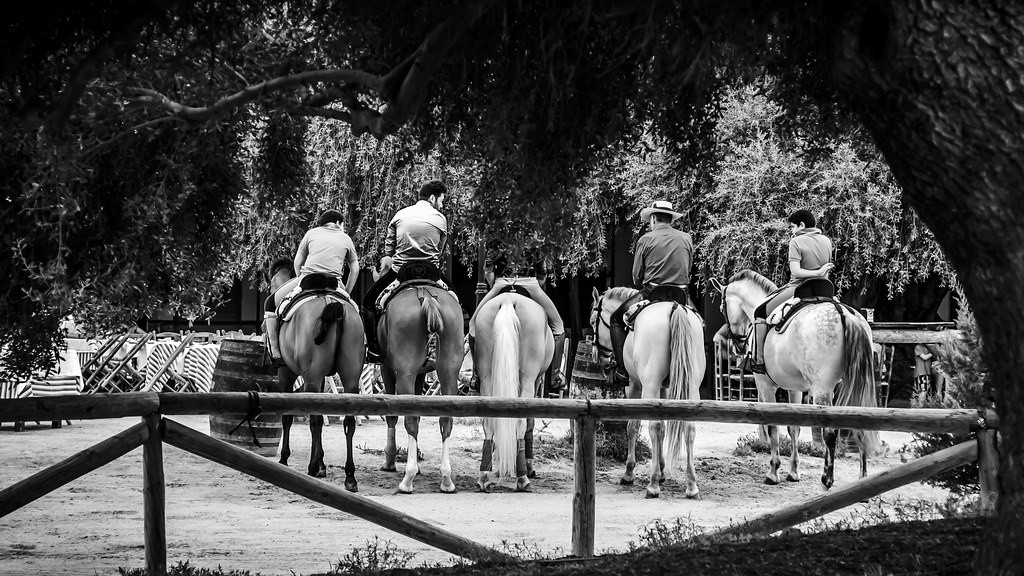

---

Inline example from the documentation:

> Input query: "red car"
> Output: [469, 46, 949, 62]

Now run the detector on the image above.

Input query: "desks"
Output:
[1, 337, 221, 394]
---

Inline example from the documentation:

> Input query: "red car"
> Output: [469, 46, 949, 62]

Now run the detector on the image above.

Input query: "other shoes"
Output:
[366, 350, 381, 363]
[270, 358, 287, 367]
[730, 361, 765, 374]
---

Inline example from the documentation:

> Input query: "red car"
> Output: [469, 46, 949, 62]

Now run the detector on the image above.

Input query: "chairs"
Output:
[76, 326, 256, 393]
[715, 340, 758, 402]
[293, 333, 475, 426]
[873, 344, 895, 408]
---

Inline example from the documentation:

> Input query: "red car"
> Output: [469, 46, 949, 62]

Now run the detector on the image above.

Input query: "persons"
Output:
[744, 209, 836, 373]
[911, 327, 933, 407]
[605, 201, 694, 386]
[263, 210, 360, 366]
[362, 181, 452, 363]
[872, 343, 888, 380]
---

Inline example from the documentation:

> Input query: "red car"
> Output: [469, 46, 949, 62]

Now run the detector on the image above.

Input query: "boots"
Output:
[605, 324, 629, 386]
[468, 334, 480, 390]
[551, 332, 567, 395]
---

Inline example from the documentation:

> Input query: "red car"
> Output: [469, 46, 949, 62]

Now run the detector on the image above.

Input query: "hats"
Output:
[640, 201, 683, 221]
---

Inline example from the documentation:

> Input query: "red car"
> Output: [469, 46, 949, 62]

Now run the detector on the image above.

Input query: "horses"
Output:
[709, 269, 875, 503]
[473, 292, 554, 492]
[264, 259, 366, 492]
[590, 287, 706, 500]
[376, 281, 464, 493]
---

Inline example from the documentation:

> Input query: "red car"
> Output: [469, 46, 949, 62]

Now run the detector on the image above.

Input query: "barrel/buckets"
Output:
[569, 340, 628, 440]
[208, 339, 291, 458]
